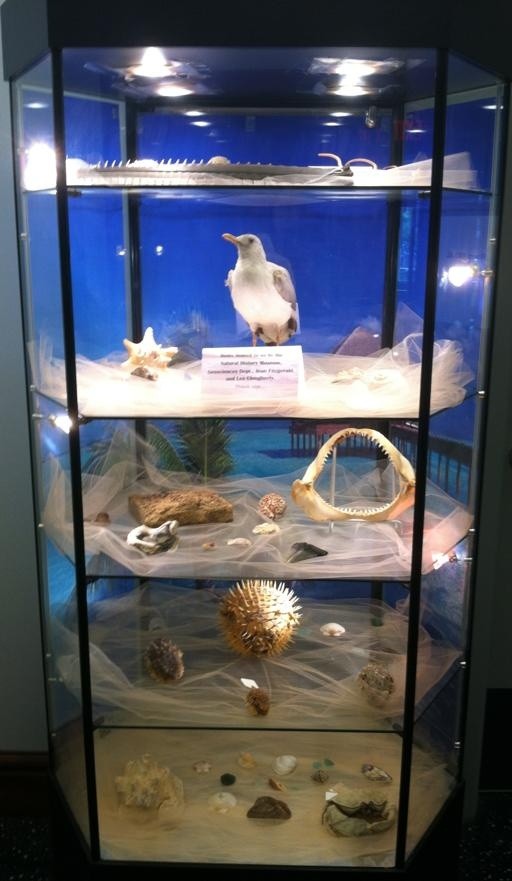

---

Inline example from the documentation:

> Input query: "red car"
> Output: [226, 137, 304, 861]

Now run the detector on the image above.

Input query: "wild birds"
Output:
[222, 231, 301, 346]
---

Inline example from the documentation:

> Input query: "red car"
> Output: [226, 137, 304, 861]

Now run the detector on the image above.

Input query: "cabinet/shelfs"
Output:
[0, 0, 512, 881]
